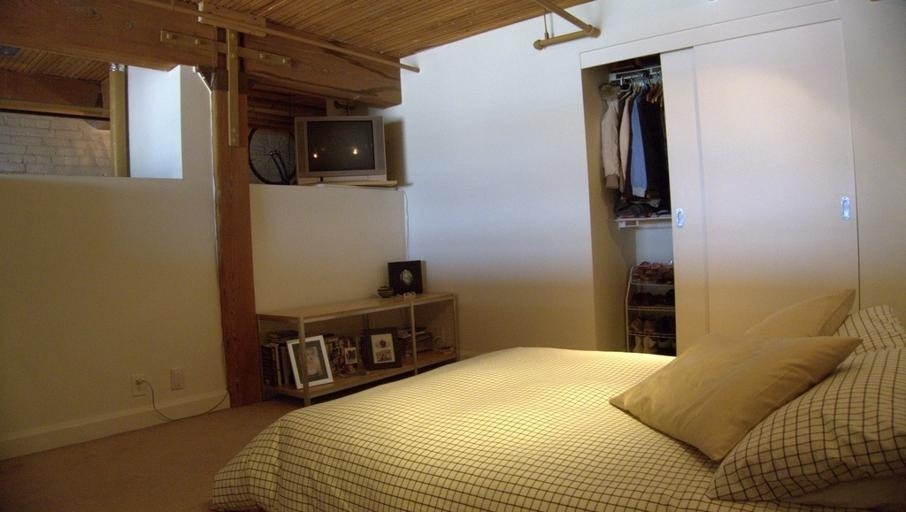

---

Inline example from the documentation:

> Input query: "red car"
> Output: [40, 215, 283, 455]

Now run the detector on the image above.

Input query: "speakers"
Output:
[387, 260, 423, 295]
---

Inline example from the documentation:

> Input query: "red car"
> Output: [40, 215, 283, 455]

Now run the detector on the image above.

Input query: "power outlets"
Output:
[132, 373, 147, 396]
[171, 368, 185, 390]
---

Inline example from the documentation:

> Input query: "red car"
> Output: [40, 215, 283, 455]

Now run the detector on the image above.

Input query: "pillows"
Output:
[784, 476, 906, 508]
[745, 289, 856, 336]
[706, 349, 906, 501]
[610, 330, 863, 462]
[831, 305, 905, 358]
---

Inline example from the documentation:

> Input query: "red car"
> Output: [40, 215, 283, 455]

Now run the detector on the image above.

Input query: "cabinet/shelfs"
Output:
[625, 266, 676, 351]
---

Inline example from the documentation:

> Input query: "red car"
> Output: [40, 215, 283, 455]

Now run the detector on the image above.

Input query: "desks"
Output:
[257, 292, 460, 407]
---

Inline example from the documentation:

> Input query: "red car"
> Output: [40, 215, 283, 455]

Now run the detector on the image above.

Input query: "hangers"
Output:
[611, 71, 663, 107]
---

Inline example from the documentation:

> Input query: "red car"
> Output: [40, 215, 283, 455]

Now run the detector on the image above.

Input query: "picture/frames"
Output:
[287, 336, 333, 389]
[365, 327, 401, 371]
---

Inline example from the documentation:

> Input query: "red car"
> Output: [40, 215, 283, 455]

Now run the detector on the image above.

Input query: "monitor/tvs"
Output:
[294, 116, 388, 183]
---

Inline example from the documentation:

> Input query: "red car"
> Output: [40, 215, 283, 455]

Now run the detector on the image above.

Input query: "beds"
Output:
[213, 347, 906, 512]
